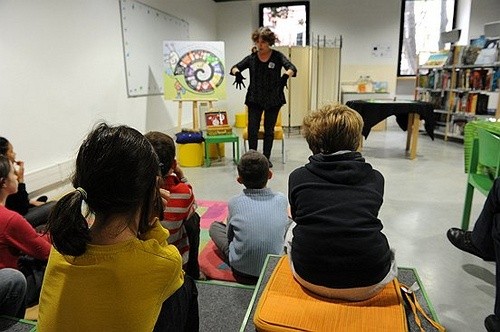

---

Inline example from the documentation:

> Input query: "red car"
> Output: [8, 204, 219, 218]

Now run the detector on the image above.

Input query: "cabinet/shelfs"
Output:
[414, 46, 500, 141]
[342, 84, 391, 131]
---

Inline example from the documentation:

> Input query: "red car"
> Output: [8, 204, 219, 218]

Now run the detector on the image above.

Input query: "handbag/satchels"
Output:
[175, 130, 205, 144]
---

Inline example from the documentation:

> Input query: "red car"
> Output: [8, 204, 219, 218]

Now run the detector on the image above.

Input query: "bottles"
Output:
[365, 75, 373, 92]
[358, 76, 364, 92]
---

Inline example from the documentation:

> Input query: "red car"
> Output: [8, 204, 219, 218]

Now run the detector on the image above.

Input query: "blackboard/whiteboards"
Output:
[119, 0, 190, 98]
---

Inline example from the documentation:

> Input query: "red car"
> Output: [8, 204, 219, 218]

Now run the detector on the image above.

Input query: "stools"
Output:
[235, 113, 246, 128]
[177, 141, 206, 167]
[203, 131, 240, 167]
[205, 142, 225, 158]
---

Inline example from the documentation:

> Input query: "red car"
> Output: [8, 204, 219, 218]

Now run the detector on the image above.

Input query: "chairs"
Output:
[461, 128, 500, 231]
[242, 104, 286, 164]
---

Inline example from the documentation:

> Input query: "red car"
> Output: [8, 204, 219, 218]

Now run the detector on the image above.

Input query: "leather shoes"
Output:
[447, 228, 491, 261]
[484, 314, 500, 332]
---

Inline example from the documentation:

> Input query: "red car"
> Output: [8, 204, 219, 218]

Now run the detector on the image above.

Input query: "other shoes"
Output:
[268, 161, 273, 168]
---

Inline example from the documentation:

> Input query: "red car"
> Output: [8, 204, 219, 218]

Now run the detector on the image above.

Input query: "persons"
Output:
[446, 177, 500, 332]
[209, 150, 289, 285]
[230, 27, 297, 168]
[0, 120, 200, 332]
[288, 103, 397, 303]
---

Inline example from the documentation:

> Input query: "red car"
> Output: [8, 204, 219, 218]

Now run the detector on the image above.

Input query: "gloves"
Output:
[233, 72, 246, 89]
[275, 74, 289, 92]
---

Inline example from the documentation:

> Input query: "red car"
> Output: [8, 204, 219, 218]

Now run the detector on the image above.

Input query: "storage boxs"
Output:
[206, 125, 233, 136]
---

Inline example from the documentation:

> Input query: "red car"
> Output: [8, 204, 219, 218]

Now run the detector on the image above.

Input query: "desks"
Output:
[346, 99, 445, 160]
